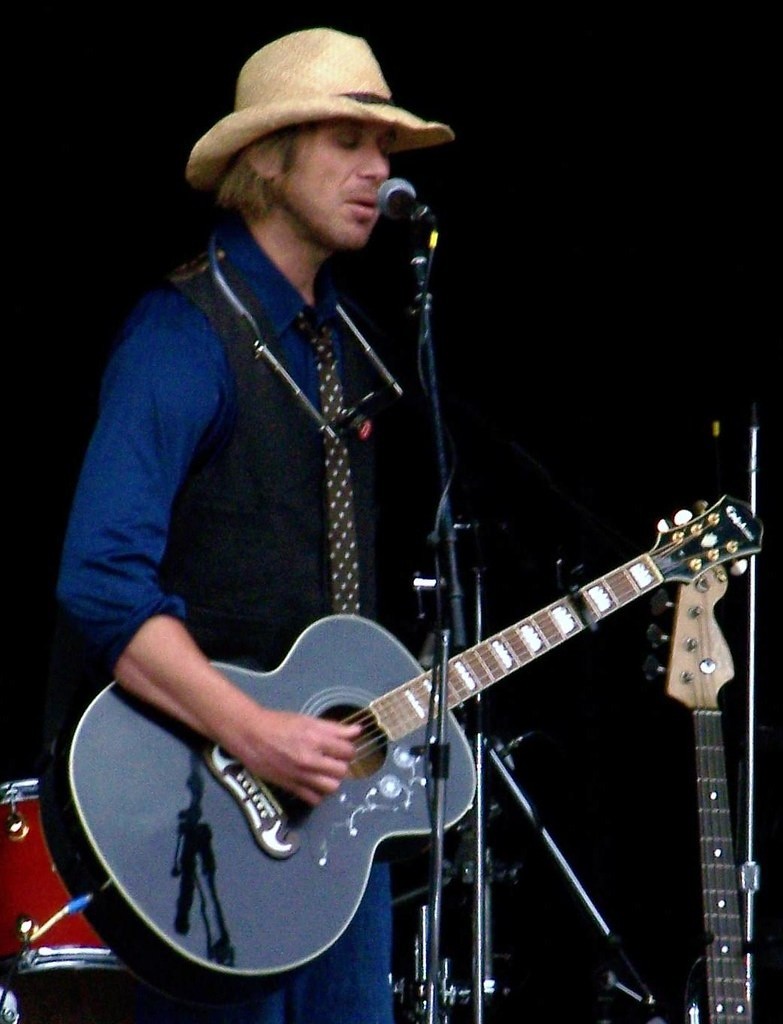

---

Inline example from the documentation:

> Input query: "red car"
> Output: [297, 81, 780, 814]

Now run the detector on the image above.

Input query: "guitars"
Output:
[37, 492, 765, 1002]
[645, 563, 755, 1023]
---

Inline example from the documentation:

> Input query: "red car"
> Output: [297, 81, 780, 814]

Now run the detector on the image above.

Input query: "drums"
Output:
[0, 778, 133, 996]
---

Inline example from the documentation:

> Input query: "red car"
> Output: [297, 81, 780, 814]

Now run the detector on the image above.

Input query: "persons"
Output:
[49, 24, 456, 1021]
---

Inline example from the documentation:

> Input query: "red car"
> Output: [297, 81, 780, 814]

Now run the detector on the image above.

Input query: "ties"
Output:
[291, 305, 361, 615]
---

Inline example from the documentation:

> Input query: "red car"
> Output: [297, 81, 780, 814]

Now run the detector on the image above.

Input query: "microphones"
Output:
[377, 177, 438, 232]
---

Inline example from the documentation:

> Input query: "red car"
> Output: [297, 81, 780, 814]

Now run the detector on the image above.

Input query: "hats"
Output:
[186, 28, 456, 191]
